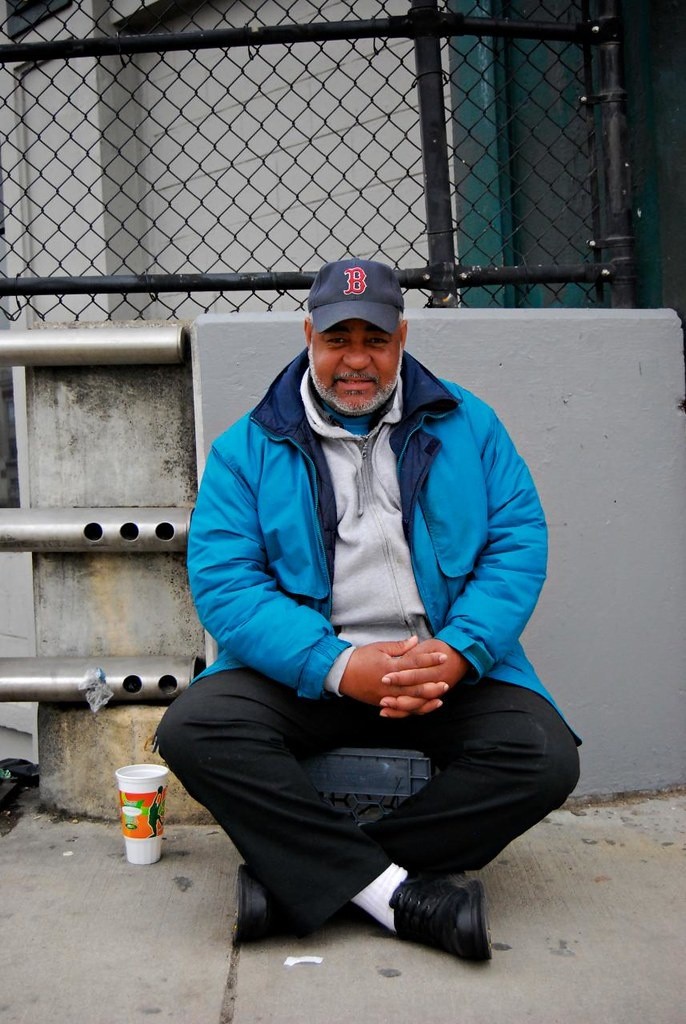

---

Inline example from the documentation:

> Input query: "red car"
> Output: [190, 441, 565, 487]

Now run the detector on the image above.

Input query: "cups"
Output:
[115, 763, 170, 864]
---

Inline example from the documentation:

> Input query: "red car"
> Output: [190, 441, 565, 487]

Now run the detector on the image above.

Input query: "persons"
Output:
[146, 261, 583, 962]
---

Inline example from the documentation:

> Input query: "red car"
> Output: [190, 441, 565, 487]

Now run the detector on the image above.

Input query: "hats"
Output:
[306, 257, 405, 335]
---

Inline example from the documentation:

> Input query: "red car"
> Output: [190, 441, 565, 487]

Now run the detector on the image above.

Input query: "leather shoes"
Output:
[388, 879, 492, 962]
[234, 862, 272, 945]
